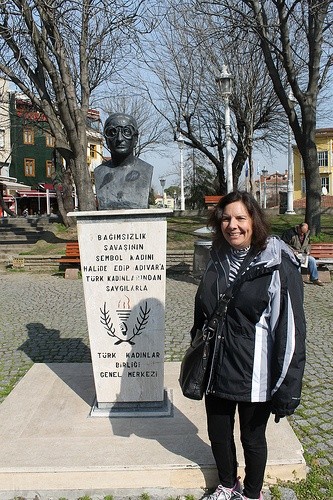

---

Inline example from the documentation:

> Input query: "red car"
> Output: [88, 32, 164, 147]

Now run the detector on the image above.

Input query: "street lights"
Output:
[173, 190, 177, 209]
[215, 64, 235, 196]
[261, 166, 268, 208]
[159, 176, 167, 208]
[176, 132, 185, 211]
[285, 84, 299, 215]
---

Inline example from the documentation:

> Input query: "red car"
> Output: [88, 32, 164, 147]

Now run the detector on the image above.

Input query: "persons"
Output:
[93, 113, 153, 211]
[22, 208, 28, 218]
[190, 190, 307, 500]
[280, 222, 324, 286]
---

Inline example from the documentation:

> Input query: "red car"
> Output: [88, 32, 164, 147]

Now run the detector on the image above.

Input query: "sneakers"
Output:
[201, 476, 243, 500]
[232, 490, 264, 500]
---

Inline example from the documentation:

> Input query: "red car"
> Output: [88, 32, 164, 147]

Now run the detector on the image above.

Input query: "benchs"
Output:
[53, 243, 80, 269]
[311, 243, 333, 264]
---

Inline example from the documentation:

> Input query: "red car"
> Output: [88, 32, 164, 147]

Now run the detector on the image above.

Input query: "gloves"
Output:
[270, 395, 302, 423]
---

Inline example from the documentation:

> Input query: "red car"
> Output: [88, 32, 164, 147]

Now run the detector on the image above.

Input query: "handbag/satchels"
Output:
[177, 248, 259, 401]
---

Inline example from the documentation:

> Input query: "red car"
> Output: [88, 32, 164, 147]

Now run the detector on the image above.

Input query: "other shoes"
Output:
[311, 279, 328, 286]
